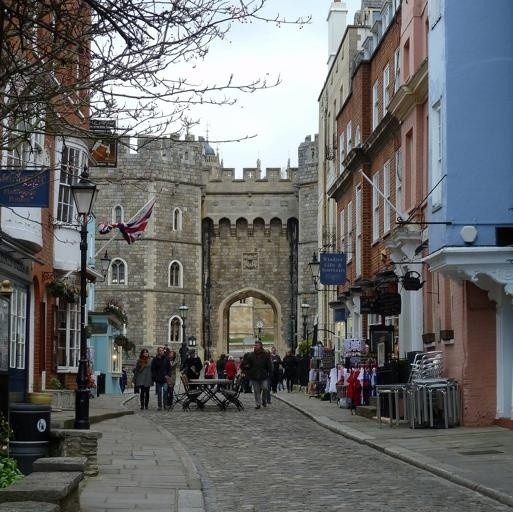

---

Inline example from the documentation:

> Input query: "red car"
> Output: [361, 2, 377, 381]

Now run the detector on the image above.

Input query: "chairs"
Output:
[165, 373, 246, 411]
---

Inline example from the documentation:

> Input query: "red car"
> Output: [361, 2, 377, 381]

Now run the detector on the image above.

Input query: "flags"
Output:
[97, 195, 160, 245]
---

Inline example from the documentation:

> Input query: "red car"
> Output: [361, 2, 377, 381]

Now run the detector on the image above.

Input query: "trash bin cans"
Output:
[8, 403, 52, 476]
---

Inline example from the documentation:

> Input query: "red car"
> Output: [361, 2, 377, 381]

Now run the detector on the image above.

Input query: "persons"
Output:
[132, 340, 310, 411]
[119, 367, 127, 394]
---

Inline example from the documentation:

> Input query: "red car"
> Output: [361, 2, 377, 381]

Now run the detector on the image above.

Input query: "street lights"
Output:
[181, 299, 186, 364]
[301, 301, 310, 339]
[70, 167, 97, 427]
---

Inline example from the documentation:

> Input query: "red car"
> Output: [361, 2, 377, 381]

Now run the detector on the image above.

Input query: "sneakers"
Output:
[255, 405, 260, 409]
[262, 400, 266, 407]
[267, 399, 270, 403]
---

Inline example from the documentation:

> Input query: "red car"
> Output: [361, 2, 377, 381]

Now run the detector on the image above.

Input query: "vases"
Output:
[28, 392, 53, 404]
